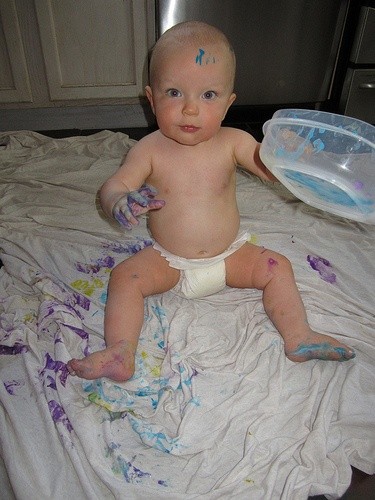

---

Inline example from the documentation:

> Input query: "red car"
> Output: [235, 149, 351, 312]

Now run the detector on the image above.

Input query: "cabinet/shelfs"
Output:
[0, 1, 155, 131]
[338, 6, 375, 121]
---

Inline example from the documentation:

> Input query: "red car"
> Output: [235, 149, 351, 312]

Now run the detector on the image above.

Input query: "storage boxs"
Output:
[258, 109, 375, 226]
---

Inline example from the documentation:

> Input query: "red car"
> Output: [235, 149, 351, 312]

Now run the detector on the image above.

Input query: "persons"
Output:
[67, 17, 356, 382]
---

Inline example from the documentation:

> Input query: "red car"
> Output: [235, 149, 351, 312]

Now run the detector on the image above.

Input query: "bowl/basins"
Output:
[259, 108, 375, 225]
